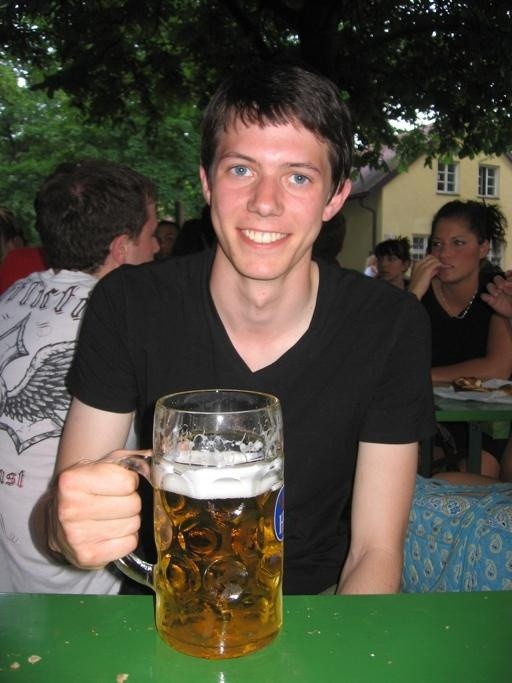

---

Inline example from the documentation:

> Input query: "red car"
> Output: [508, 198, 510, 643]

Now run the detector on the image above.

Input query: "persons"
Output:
[0, 158, 512, 594]
[44, 66, 434, 594]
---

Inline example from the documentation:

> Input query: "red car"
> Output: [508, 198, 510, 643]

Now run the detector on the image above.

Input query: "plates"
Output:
[434, 384, 512, 406]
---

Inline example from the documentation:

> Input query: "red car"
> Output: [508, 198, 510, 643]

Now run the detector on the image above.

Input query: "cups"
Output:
[106, 386, 289, 661]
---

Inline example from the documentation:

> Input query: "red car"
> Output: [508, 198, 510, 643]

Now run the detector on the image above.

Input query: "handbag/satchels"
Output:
[401, 474, 511, 593]
